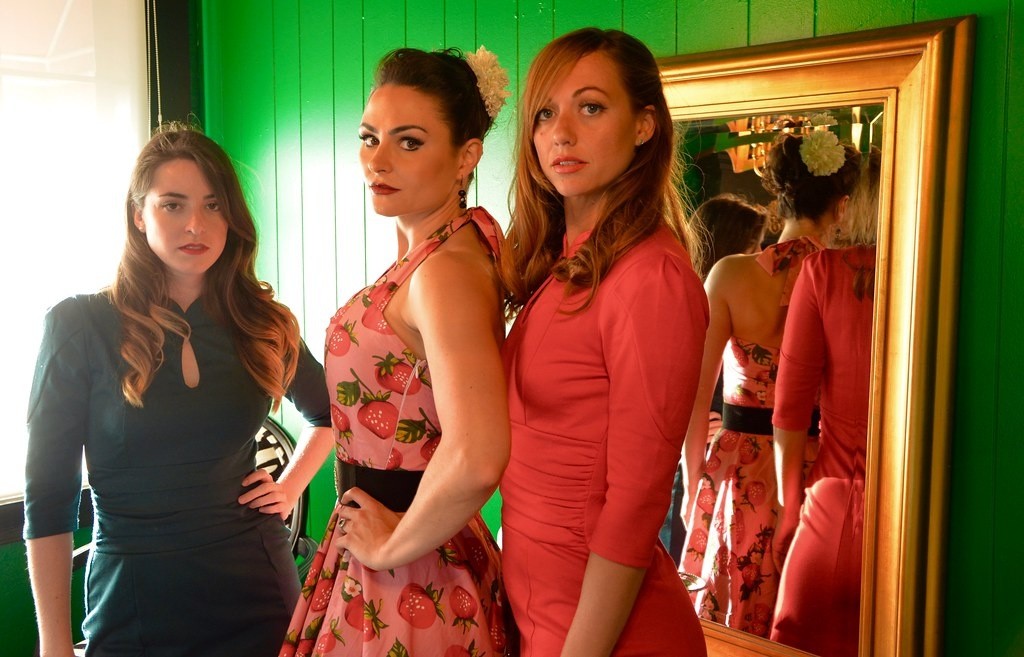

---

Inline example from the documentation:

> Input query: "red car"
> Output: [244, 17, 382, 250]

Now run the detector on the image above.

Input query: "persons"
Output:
[502, 27, 707, 657]
[279, 44, 512, 657]
[665, 192, 766, 450]
[772, 147, 881, 657]
[676, 132, 861, 638]
[21, 120, 336, 657]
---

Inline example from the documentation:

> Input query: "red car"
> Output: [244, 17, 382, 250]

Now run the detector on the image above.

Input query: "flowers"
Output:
[800, 130, 845, 177]
[463, 43, 512, 122]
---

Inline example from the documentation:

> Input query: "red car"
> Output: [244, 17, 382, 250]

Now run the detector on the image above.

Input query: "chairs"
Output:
[69, 416, 318, 656]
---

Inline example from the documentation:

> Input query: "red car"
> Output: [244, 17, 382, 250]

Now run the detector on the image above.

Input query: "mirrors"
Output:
[659, 12, 979, 657]
